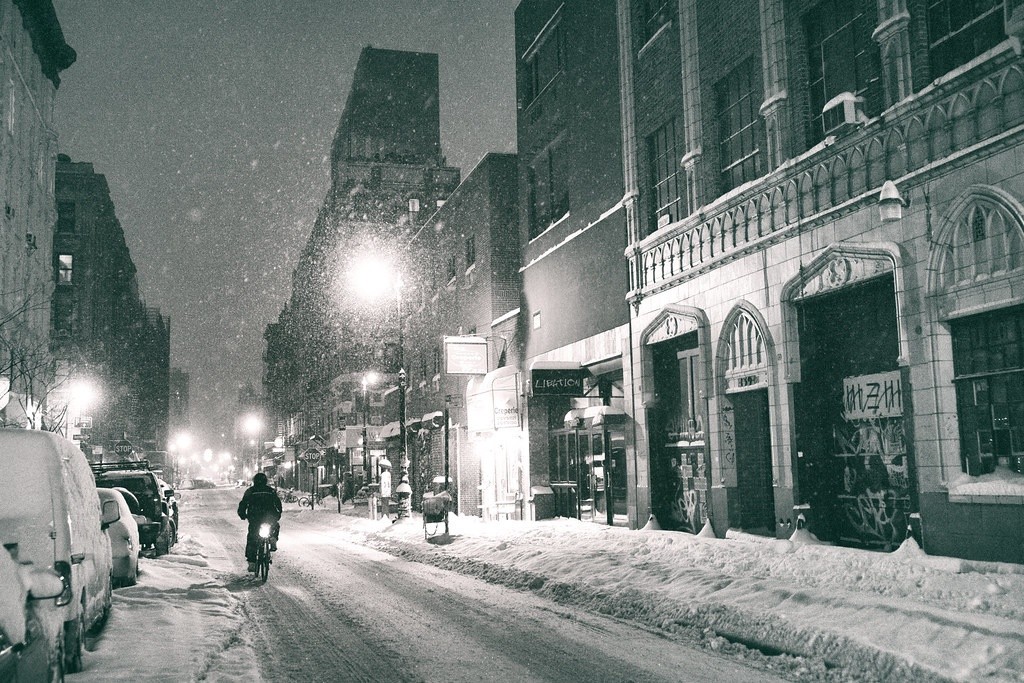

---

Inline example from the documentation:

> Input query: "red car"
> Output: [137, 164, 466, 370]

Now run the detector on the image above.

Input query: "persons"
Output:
[237, 473, 282, 572]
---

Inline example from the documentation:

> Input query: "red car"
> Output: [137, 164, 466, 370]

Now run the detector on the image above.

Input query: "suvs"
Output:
[90, 460, 178, 555]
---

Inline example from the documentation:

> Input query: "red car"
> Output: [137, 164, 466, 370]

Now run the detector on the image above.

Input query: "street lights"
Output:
[356, 261, 413, 522]
[362, 371, 378, 484]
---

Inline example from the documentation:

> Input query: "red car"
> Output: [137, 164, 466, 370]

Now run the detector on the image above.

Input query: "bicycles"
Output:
[277, 486, 298, 503]
[242, 505, 273, 582]
[299, 491, 324, 508]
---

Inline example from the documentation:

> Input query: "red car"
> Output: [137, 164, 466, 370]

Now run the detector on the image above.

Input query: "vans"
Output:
[0, 427, 113, 675]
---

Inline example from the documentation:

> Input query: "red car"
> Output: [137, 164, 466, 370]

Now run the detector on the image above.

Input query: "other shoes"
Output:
[269, 538, 278, 551]
[248, 560, 256, 572]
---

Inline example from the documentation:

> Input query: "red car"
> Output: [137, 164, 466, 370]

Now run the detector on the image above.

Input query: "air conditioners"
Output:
[5, 206, 15, 220]
[822, 100, 863, 136]
[26, 232, 36, 246]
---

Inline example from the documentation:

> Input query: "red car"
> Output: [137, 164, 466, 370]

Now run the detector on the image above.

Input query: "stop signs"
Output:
[115, 439, 133, 458]
[303, 447, 321, 466]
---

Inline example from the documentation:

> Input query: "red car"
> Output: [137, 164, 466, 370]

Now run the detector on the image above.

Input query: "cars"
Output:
[94, 486, 144, 587]
[0, 544, 69, 683]
